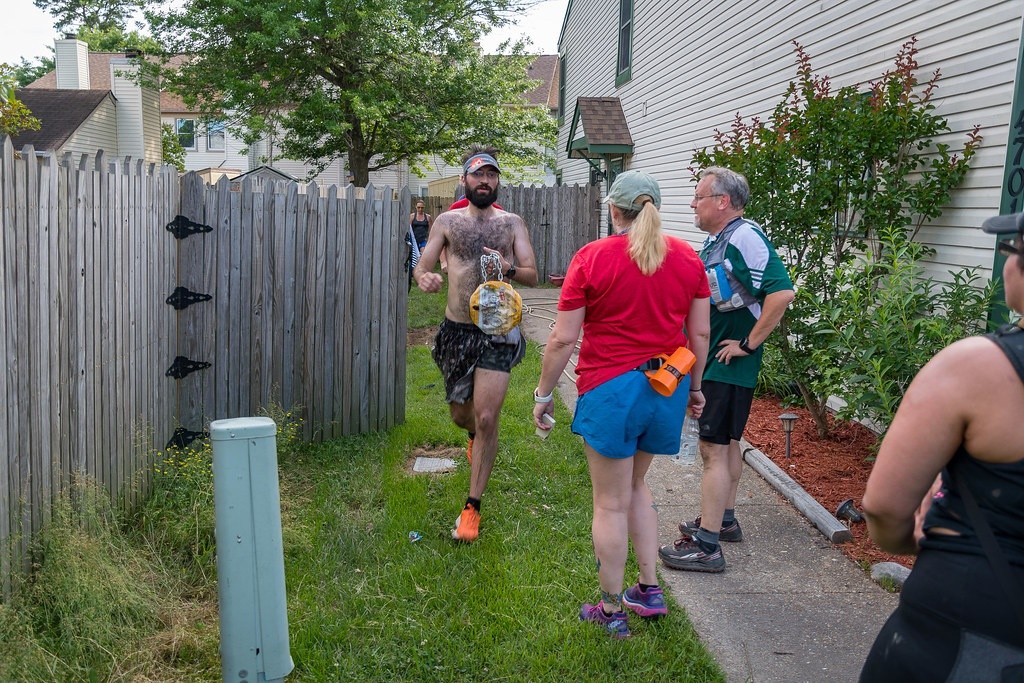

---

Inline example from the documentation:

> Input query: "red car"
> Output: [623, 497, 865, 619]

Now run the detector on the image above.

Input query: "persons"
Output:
[533, 170, 712, 639]
[658, 166, 794, 572]
[860, 209, 1024, 683]
[413, 154, 539, 543]
[409, 147, 529, 274]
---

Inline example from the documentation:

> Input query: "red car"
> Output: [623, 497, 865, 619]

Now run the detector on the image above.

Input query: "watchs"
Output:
[503, 264, 515, 279]
[739, 337, 759, 354]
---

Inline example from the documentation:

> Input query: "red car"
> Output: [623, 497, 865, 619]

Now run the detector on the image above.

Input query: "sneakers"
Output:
[578, 601, 631, 640]
[467, 436, 475, 465]
[451, 503, 482, 542]
[659, 535, 727, 574]
[622, 582, 668, 622]
[678, 514, 743, 542]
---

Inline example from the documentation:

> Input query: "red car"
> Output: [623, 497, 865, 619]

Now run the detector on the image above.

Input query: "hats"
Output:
[603, 170, 662, 211]
[463, 153, 502, 174]
[981, 209, 1024, 235]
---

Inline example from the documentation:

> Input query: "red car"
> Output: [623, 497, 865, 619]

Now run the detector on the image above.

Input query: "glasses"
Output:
[474, 170, 500, 178]
[998, 239, 1024, 257]
[693, 192, 733, 204]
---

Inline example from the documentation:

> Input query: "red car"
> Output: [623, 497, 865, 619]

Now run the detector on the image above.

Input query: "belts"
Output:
[631, 356, 674, 372]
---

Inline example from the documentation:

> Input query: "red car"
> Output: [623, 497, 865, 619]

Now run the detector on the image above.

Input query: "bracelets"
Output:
[534, 387, 552, 402]
[690, 389, 701, 392]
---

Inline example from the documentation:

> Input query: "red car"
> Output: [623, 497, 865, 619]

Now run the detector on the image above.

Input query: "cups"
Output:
[644, 347, 696, 396]
